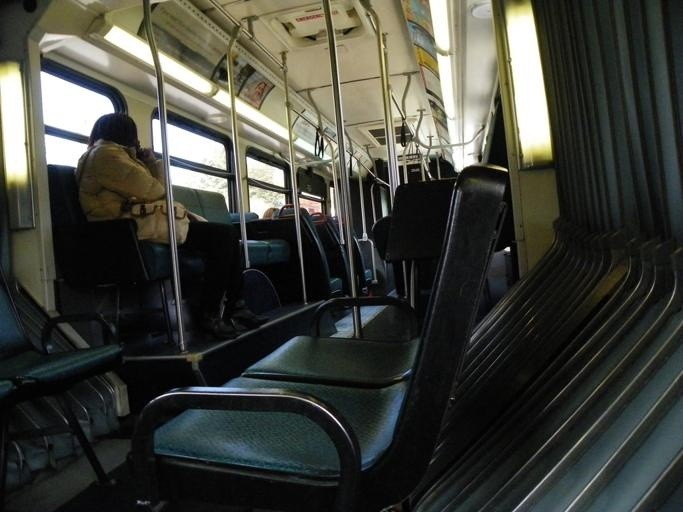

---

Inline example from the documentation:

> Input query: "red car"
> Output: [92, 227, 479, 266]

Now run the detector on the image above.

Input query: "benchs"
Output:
[0, 166, 512, 511]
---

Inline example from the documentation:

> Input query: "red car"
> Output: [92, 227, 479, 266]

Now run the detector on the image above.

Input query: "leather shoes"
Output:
[196, 308, 241, 340]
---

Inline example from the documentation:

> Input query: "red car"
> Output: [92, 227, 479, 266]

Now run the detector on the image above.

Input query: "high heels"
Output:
[223, 299, 271, 330]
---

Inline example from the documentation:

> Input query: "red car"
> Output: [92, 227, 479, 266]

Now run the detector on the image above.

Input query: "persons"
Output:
[75, 109, 269, 346]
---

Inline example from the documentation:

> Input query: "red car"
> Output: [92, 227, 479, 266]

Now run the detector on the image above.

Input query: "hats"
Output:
[88, 114, 140, 147]
